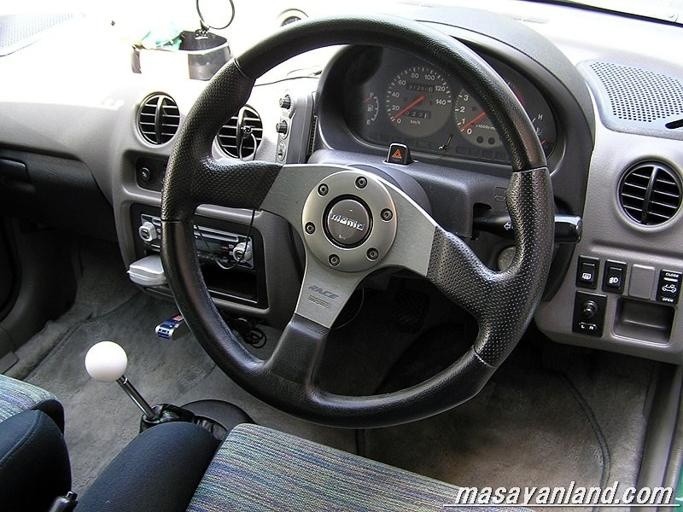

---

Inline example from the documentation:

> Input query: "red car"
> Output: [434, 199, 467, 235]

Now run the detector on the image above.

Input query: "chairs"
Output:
[70, 418, 534, 511]
[0, 370, 72, 512]
